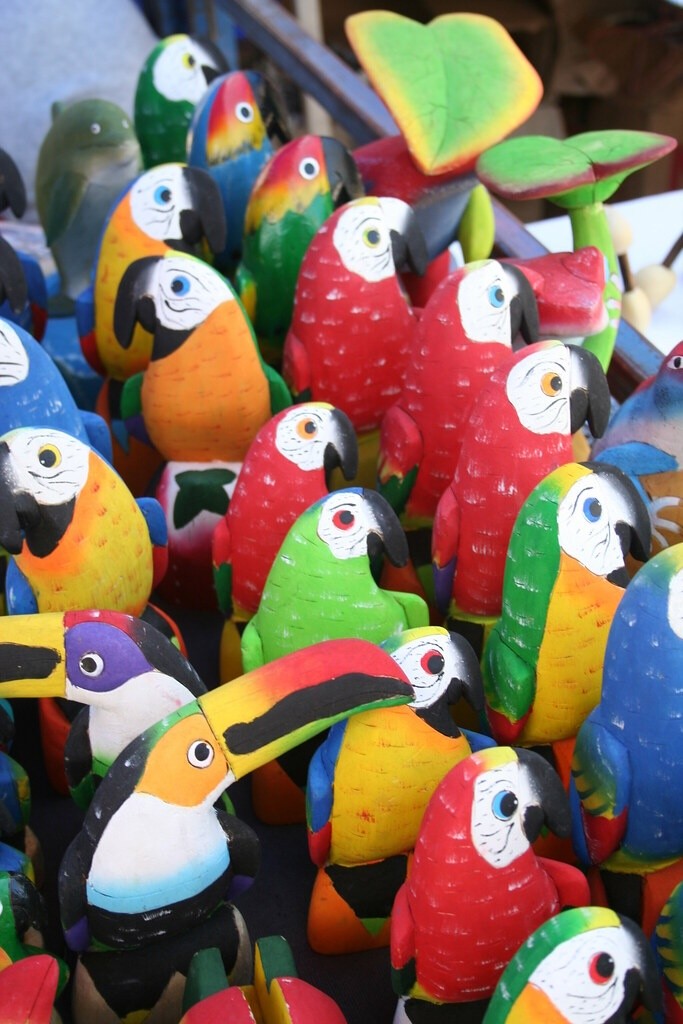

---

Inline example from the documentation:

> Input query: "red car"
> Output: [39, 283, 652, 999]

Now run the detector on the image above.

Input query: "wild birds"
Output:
[0, 611, 415, 1024]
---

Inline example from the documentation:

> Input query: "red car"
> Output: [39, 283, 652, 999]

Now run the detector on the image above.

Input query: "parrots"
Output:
[0, 34, 683, 1024]
